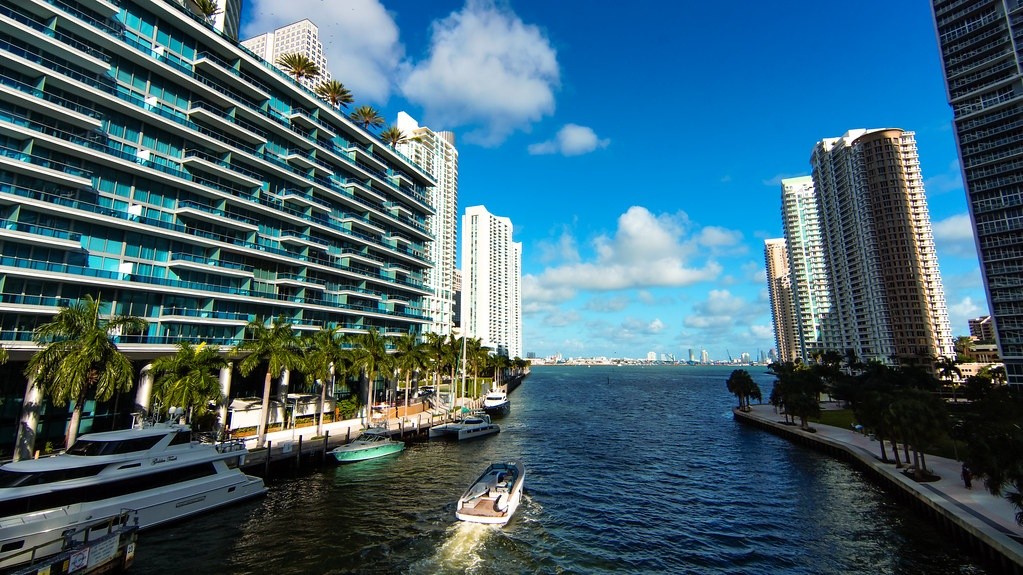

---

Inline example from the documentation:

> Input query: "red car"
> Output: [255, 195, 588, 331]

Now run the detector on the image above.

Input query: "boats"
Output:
[428, 412, 501, 441]
[0, 406, 265, 575]
[483, 382, 511, 418]
[453, 453, 527, 525]
[328, 425, 406, 464]
